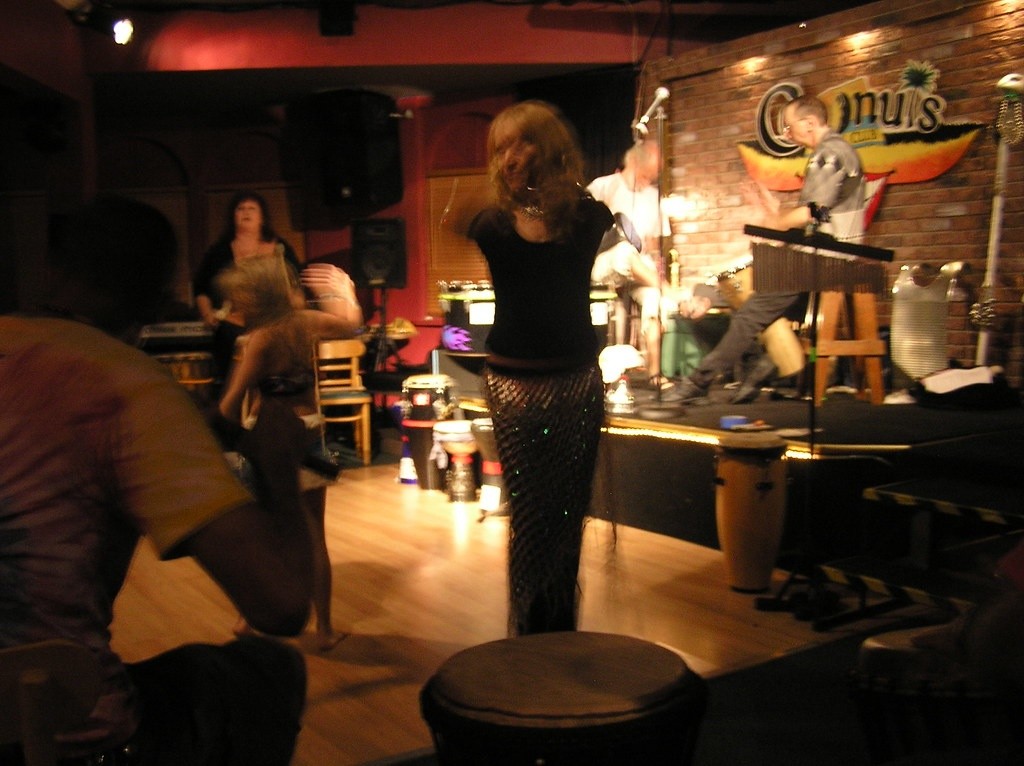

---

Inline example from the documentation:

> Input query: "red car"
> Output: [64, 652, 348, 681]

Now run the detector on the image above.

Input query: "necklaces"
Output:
[520, 202, 543, 222]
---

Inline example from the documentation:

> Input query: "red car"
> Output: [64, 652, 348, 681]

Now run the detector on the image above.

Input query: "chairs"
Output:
[311, 336, 372, 465]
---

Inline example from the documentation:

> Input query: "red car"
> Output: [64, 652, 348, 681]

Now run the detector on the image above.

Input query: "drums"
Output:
[392, 373, 510, 517]
[707, 253, 809, 377]
[713, 433, 791, 592]
[418, 630, 714, 766]
[592, 211, 642, 281]
[851, 609, 1024, 766]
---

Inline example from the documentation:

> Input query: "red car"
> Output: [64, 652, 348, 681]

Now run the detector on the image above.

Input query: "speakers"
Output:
[349, 217, 407, 289]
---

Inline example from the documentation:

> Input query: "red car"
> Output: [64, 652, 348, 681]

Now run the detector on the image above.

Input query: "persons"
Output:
[441, 100, 613, 641]
[580, 140, 710, 392]
[659, 94, 867, 405]
[0, 190, 364, 766]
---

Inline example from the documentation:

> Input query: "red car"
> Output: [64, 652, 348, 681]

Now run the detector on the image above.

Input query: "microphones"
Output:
[640, 86, 670, 124]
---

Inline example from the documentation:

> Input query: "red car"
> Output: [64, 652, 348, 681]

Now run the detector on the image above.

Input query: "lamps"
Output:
[67, 4, 133, 45]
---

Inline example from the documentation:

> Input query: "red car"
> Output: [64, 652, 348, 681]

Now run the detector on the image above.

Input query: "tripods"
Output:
[361, 288, 411, 392]
